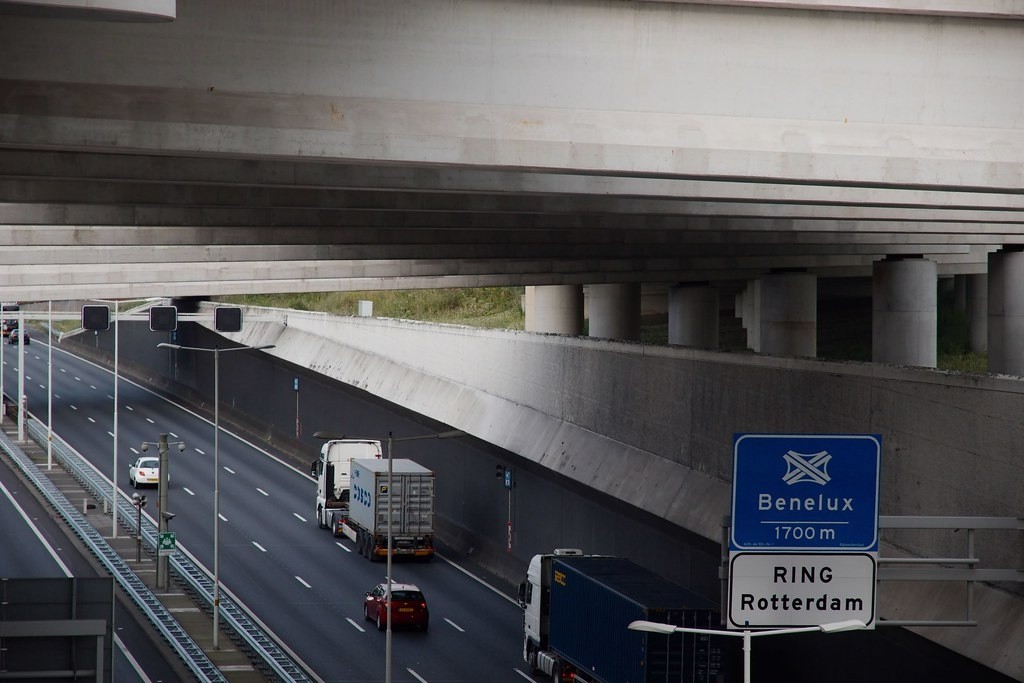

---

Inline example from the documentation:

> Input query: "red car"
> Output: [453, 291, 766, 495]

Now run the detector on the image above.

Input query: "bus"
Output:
[0, 302, 19, 337]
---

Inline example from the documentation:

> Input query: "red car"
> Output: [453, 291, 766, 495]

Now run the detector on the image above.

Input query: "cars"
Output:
[9, 329, 30, 345]
[364, 583, 429, 631]
[129, 457, 170, 489]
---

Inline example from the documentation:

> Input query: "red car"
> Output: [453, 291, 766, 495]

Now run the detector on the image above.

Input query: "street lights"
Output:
[157, 342, 276, 651]
[89, 298, 163, 538]
[142, 432, 185, 589]
[313, 431, 466, 683]
[628, 619, 867, 683]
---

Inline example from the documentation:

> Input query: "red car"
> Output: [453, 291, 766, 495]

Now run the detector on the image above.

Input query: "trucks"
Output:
[312, 439, 436, 563]
[518, 548, 745, 683]
